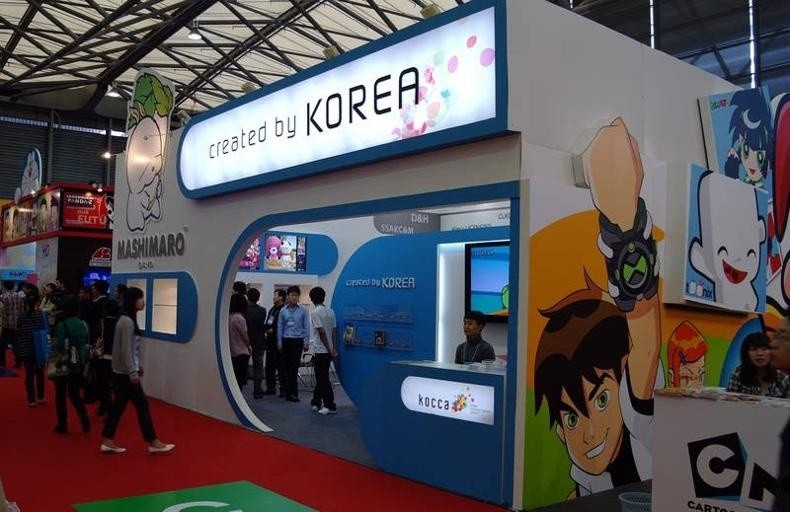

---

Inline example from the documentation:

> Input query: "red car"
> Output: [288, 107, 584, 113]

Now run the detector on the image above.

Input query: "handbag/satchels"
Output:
[89, 336, 104, 360]
[48, 346, 80, 383]
[33, 328, 52, 369]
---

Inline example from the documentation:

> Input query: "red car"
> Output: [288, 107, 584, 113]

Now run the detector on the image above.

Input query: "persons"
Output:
[228, 281, 337, 416]
[728, 332, 789, 397]
[1, 279, 175, 454]
[454, 309, 495, 365]
[770, 308, 789, 512]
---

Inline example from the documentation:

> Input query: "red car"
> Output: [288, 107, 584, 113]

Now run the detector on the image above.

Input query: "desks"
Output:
[649, 386, 789, 511]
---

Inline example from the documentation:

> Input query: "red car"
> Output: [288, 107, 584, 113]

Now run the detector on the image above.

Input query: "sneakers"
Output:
[312, 405, 336, 415]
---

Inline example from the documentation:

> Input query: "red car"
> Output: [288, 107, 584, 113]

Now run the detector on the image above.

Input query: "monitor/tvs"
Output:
[464, 241, 510, 324]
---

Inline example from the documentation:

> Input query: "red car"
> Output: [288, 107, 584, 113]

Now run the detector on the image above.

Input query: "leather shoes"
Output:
[101, 444, 126, 453]
[149, 444, 175, 453]
[285, 394, 299, 402]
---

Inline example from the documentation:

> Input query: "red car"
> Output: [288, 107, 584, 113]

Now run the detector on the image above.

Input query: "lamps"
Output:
[143, 131, 173, 141]
[187, 17, 201, 41]
[100, 149, 117, 158]
[96, 182, 105, 193]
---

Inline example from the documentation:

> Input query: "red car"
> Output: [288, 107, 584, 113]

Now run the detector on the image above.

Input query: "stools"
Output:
[298, 354, 316, 391]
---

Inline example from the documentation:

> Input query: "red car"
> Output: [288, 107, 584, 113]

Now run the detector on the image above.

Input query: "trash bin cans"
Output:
[619, 492, 652, 512]
